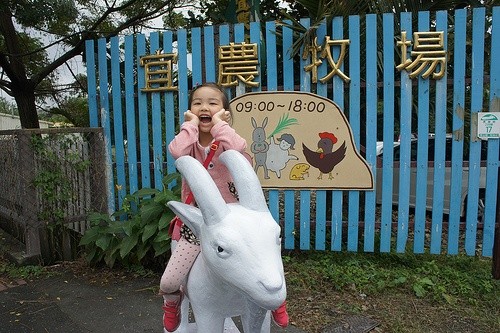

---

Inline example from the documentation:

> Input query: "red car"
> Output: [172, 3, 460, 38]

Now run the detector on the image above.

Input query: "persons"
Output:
[161, 81, 290, 332]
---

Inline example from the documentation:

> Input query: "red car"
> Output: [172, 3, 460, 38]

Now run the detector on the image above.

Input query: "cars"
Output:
[374, 133, 500, 225]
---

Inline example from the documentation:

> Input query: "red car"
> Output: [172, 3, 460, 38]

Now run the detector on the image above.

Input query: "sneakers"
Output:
[163, 291, 185, 332]
[270, 300, 290, 328]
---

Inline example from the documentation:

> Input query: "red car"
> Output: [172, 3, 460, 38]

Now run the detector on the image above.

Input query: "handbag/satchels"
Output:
[169, 216, 183, 241]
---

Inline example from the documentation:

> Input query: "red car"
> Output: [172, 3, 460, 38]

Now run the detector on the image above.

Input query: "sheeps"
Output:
[163, 149, 288, 333]
